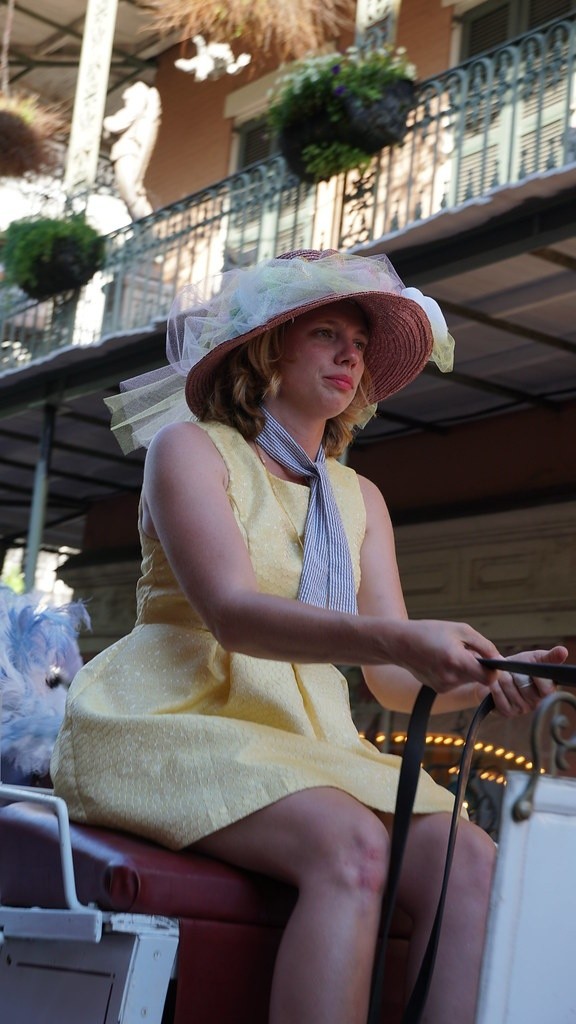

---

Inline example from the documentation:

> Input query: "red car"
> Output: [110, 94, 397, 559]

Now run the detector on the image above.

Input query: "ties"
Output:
[250, 397, 363, 618]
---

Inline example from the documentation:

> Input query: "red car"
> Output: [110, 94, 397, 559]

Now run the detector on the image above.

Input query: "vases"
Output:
[273, 73, 421, 185]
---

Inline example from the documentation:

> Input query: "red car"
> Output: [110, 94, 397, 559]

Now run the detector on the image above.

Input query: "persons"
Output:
[50, 249, 567, 1022]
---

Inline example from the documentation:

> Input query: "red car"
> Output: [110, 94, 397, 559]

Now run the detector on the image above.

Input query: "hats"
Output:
[186, 249, 436, 420]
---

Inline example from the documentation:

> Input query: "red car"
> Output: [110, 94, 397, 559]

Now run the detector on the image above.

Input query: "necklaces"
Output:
[253, 440, 305, 556]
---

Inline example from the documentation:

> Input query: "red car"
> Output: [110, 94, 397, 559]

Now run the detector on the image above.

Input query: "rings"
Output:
[517, 681, 534, 690]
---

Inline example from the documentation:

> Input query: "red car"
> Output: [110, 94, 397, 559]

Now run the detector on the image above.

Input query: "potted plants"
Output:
[0, 217, 108, 301]
[0, 88, 68, 179]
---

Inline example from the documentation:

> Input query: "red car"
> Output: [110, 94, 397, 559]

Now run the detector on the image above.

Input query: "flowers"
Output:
[261, 44, 419, 140]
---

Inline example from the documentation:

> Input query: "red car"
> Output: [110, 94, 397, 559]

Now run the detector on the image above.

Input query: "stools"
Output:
[2, 787, 301, 1024]
